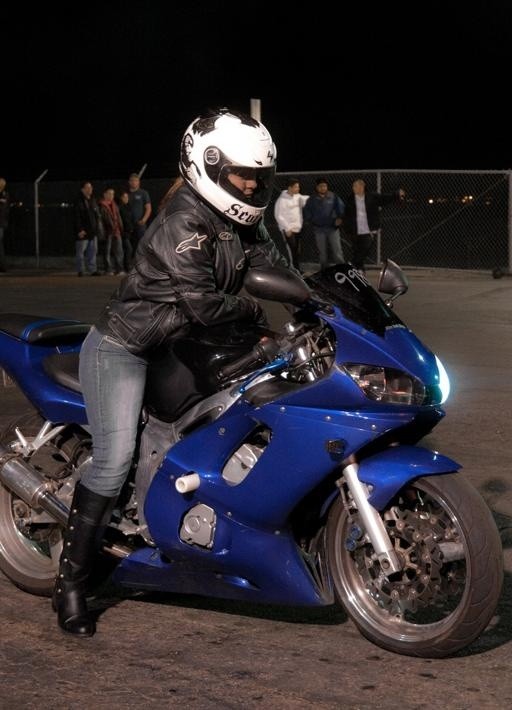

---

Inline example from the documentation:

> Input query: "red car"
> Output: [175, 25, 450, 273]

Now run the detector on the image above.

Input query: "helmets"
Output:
[176, 106, 280, 227]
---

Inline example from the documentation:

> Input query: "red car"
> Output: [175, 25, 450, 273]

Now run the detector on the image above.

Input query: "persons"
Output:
[68, 179, 104, 278]
[302, 177, 347, 271]
[125, 171, 151, 251]
[116, 190, 137, 274]
[51, 105, 309, 639]
[273, 177, 311, 278]
[333, 178, 407, 270]
[96, 186, 126, 278]
[0, 177, 11, 273]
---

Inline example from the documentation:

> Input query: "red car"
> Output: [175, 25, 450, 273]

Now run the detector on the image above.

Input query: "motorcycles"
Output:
[0, 257, 506, 664]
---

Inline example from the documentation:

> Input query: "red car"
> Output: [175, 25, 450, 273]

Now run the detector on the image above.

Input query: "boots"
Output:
[52, 479, 118, 636]
